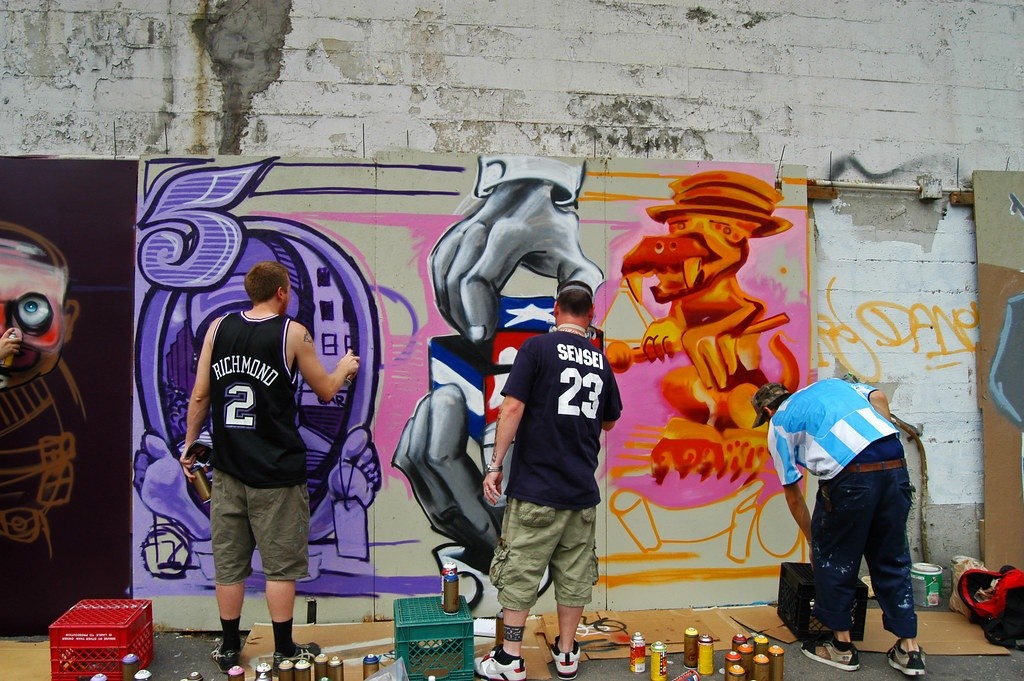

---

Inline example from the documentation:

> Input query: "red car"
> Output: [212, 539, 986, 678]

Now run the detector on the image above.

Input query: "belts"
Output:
[849, 458, 907, 473]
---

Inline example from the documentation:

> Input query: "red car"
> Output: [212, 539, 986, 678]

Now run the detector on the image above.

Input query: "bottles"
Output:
[255, 654, 344, 681]
[697, 634, 714, 675]
[683, 627, 699, 670]
[724, 633, 785, 681]
[90, 674, 108, 681]
[187, 671, 204, 681]
[630, 632, 646, 673]
[190, 463, 213, 504]
[122, 654, 152, 681]
[363, 654, 379, 680]
[0, 331, 17, 367]
[441, 561, 460, 615]
[650, 641, 667, 681]
[227, 665, 245, 681]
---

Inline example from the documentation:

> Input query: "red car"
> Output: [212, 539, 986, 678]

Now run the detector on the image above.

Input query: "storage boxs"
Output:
[393, 595, 475, 681]
[777, 562, 869, 642]
[49, 600, 154, 681]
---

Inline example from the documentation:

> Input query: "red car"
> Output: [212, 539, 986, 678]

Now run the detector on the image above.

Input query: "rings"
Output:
[485, 489, 490, 492]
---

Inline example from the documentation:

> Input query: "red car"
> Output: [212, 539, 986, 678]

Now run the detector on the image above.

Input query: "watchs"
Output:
[486, 464, 503, 472]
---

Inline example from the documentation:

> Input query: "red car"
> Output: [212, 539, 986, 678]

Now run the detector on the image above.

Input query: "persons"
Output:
[753, 378, 927, 676]
[179, 260, 362, 673]
[472, 280, 624, 681]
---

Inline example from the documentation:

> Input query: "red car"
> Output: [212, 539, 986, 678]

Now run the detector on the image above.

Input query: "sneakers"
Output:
[886, 637, 926, 675]
[473, 644, 526, 681]
[801, 638, 861, 671]
[550, 631, 580, 680]
[271, 643, 322, 676]
[210, 642, 240, 674]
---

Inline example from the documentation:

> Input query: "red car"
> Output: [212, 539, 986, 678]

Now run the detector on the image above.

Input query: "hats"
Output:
[753, 384, 790, 428]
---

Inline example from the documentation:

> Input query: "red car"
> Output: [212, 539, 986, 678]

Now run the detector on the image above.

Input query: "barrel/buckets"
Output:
[951, 556, 981, 593]
[909, 562, 943, 608]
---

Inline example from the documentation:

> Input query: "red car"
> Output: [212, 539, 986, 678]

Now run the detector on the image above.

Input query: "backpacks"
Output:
[958, 566, 1024, 652]
